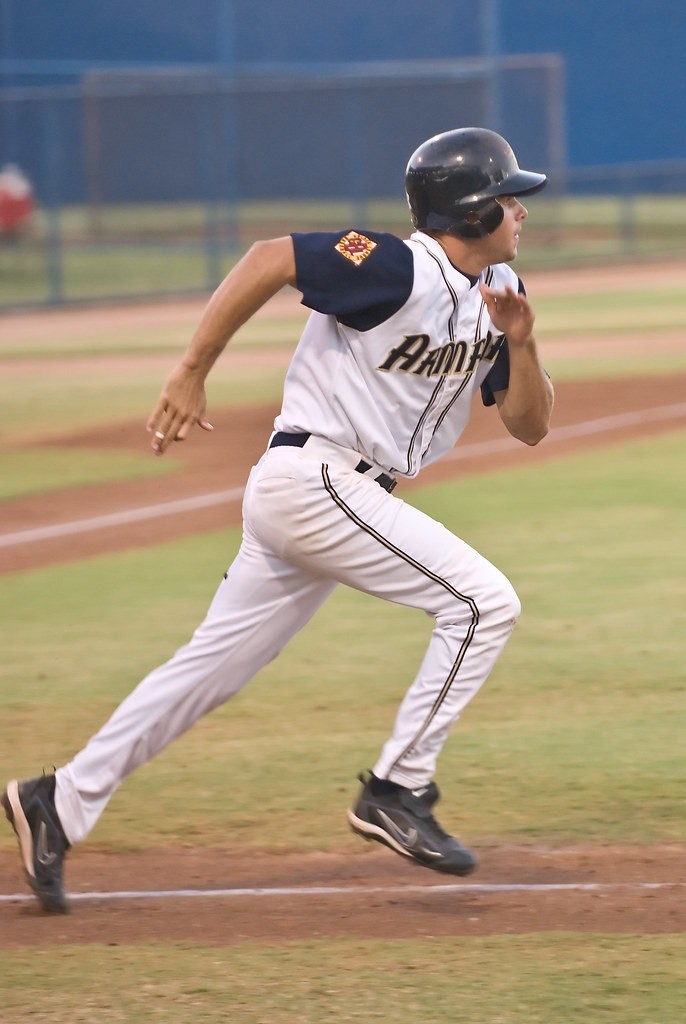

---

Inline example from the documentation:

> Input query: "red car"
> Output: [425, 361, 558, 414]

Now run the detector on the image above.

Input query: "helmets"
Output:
[404, 126, 547, 240]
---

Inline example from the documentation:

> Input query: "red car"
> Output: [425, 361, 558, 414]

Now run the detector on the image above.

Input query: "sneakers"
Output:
[3, 763, 71, 914]
[345, 768, 476, 879]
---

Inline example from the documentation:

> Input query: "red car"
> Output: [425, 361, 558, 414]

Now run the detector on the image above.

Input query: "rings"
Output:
[155, 432, 164, 440]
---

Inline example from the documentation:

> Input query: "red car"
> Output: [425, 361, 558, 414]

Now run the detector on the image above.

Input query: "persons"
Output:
[0, 128, 554, 914]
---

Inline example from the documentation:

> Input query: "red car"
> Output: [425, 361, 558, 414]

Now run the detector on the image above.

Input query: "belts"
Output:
[269, 429, 398, 494]
